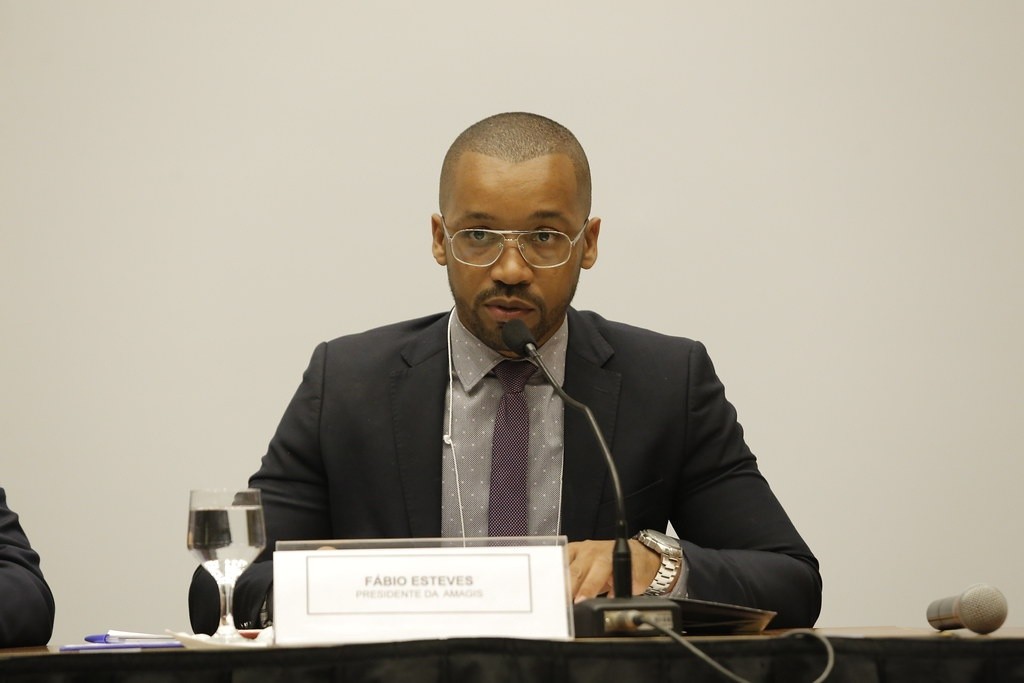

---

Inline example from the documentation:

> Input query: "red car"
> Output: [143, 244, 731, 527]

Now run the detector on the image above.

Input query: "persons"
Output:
[233, 110, 824, 643]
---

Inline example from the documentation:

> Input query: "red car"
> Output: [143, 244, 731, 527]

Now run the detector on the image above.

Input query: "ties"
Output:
[487, 359, 538, 537]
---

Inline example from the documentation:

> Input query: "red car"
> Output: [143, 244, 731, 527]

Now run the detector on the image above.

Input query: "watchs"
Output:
[629, 529, 684, 598]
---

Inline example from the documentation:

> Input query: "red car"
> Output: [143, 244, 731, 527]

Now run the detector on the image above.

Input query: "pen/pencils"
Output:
[84, 634, 180, 645]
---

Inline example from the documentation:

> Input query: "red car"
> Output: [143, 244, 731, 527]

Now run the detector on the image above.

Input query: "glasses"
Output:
[441, 216, 589, 269]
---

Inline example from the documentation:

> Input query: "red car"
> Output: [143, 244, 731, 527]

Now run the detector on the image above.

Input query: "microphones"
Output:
[925, 585, 1007, 635]
[500, 320, 684, 636]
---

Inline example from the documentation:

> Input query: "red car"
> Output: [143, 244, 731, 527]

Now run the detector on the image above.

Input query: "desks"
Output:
[1, 624, 1023, 682]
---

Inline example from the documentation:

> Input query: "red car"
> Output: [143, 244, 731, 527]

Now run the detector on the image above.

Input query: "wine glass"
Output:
[187, 488, 269, 646]
